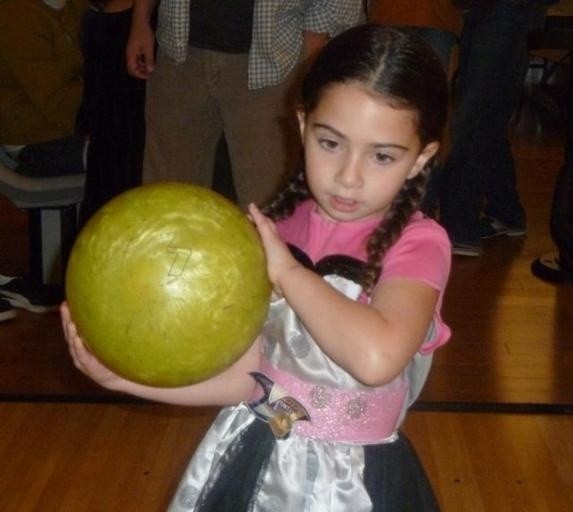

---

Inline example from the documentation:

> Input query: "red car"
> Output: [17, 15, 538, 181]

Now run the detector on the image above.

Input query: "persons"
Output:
[58, 23, 453, 512]
[0, 1, 363, 323]
[432, 0, 572, 285]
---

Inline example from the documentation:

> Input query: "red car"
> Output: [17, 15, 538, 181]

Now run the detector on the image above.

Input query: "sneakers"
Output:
[476, 216, 526, 240]
[450, 238, 480, 258]
[0, 269, 70, 325]
